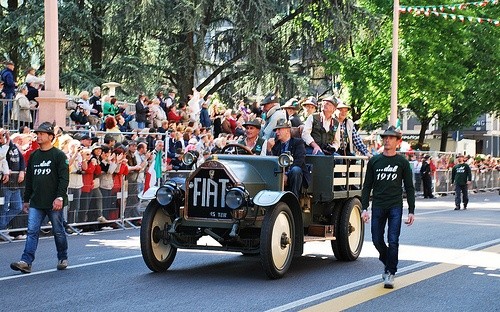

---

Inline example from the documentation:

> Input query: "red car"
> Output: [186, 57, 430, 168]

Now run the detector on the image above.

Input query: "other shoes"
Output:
[0, 233, 14, 240]
[14, 235, 27, 239]
[454, 206, 459, 210]
[57, 259, 68, 270]
[382, 264, 390, 280]
[383, 272, 394, 289]
[97, 216, 108, 222]
[10, 261, 32, 273]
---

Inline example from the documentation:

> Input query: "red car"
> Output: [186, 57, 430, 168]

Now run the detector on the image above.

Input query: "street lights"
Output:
[401, 108, 410, 130]
[105, 81, 120, 97]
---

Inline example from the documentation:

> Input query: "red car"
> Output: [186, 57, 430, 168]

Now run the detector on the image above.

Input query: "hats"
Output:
[231, 110, 237, 115]
[380, 126, 402, 138]
[272, 118, 291, 132]
[456, 153, 464, 158]
[168, 89, 175, 93]
[425, 155, 431, 158]
[90, 142, 104, 154]
[242, 119, 261, 129]
[33, 121, 55, 140]
[280, 98, 302, 109]
[260, 92, 278, 105]
[27, 67, 35, 72]
[134, 137, 148, 144]
[7, 60, 15, 65]
[301, 98, 318, 108]
[336, 102, 351, 110]
[323, 95, 338, 106]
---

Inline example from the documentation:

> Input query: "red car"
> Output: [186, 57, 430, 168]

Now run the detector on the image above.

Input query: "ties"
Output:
[282, 143, 286, 151]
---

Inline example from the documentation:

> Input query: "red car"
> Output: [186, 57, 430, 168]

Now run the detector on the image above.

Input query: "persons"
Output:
[0, 61, 369, 242]
[369, 143, 500, 199]
[10, 122, 69, 274]
[361, 125, 415, 288]
[451, 152, 472, 210]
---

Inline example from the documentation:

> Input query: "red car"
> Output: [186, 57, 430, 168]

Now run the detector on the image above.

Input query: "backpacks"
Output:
[159, 97, 174, 111]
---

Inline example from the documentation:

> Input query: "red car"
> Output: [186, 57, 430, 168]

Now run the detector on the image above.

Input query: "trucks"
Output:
[140, 143, 370, 280]
